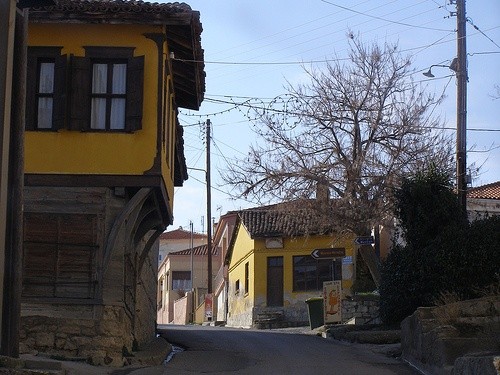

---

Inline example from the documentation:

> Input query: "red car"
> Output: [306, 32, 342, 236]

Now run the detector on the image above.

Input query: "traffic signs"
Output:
[310, 248, 346, 260]
[354, 236, 375, 244]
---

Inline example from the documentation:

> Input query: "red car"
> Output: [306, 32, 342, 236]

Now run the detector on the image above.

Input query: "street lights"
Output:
[424, 0, 468, 216]
[188, 120, 212, 296]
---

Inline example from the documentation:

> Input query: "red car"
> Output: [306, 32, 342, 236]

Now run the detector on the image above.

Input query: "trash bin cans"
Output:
[304, 296, 325, 331]
